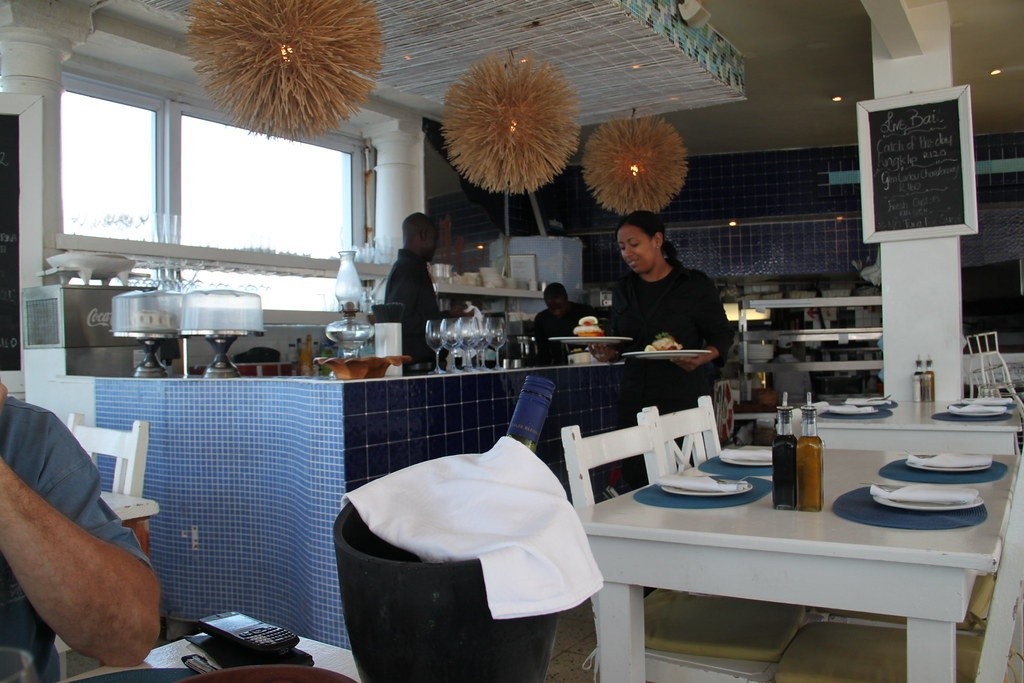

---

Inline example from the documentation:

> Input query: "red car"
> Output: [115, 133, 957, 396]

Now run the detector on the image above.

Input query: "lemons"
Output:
[644, 345, 657, 352]
[578, 316, 598, 324]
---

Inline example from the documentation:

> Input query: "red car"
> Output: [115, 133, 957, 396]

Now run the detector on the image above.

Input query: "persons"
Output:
[588, 210, 736, 484]
[0, 376, 160, 683]
[385, 212, 461, 371]
[533, 283, 598, 364]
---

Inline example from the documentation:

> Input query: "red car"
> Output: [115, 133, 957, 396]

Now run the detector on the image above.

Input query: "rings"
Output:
[601, 354, 606, 356]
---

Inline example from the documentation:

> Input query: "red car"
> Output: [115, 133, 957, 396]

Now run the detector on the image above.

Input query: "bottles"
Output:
[913, 360, 924, 402]
[772, 405, 797, 511]
[923, 360, 935, 402]
[797, 405, 824, 511]
[287, 334, 334, 377]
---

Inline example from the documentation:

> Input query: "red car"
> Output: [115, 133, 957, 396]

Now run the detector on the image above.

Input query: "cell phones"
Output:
[198, 611, 300, 656]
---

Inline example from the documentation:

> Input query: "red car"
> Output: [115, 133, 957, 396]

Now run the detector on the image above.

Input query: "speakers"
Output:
[678, 0, 711, 29]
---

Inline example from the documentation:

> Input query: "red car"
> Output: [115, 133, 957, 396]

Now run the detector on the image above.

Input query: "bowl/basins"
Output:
[170, 663, 359, 683]
[460, 263, 517, 290]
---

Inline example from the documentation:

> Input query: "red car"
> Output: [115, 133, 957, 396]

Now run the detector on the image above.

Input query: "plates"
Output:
[549, 336, 633, 344]
[739, 342, 774, 364]
[829, 397, 891, 415]
[947, 397, 1014, 417]
[621, 349, 713, 359]
[44, 251, 133, 278]
[720, 445, 780, 466]
[660, 477, 753, 497]
[872, 488, 984, 511]
[906, 453, 992, 471]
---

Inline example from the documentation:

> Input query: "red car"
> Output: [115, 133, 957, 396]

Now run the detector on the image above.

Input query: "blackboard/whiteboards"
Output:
[856, 83, 978, 243]
[0, 91, 44, 400]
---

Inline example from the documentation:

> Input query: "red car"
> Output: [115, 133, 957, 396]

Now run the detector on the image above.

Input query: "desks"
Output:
[575, 449, 1020, 683]
[53, 631, 362, 683]
[799, 397, 1021, 456]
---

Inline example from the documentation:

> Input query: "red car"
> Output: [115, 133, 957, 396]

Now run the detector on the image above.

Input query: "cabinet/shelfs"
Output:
[742, 295, 882, 374]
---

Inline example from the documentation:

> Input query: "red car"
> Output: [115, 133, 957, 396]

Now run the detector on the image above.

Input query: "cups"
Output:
[0, 647, 41, 682]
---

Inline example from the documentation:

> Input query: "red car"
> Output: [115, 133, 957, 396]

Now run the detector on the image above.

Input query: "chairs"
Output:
[66, 412, 149, 496]
[558, 393, 1024, 683]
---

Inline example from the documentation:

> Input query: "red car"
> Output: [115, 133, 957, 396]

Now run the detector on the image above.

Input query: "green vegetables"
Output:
[655, 332, 674, 339]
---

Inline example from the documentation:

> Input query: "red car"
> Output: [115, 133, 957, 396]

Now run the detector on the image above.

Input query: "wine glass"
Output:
[62, 198, 155, 241]
[425, 320, 448, 375]
[486, 317, 506, 371]
[126, 256, 282, 304]
[440, 317, 487, 374]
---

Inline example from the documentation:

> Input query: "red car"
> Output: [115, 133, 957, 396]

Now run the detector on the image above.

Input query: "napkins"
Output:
[825, 405, 875, 412]
[869, 483, 978, 505]
[906, 450, 993, 468]
[656, 473, 746, 492]
[954, 397, 1013, 406]
[719, 447, 772, 460]
[846, 397, 894, 406]
[949, 404, 1007, 413]
